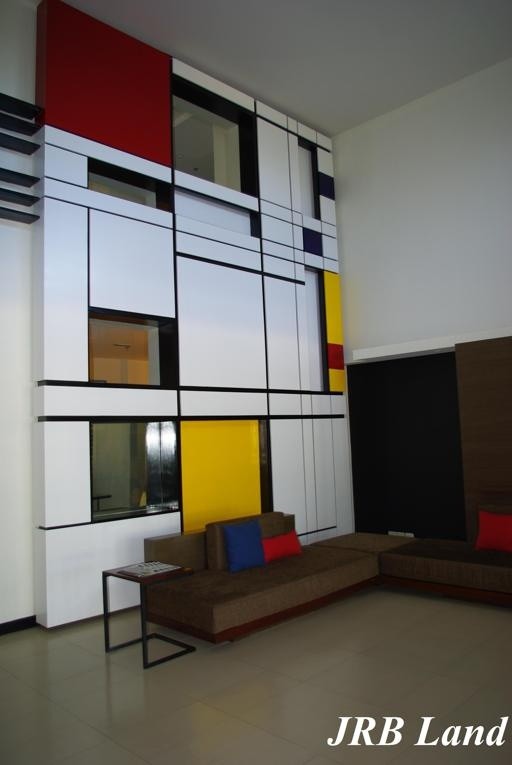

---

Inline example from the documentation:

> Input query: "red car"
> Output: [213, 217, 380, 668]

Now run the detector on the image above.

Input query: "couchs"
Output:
[143, 501, 512, 647]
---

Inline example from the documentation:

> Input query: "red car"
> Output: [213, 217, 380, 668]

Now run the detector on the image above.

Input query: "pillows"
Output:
[475, 509, 512, 552]
[221, 519, 303, 573]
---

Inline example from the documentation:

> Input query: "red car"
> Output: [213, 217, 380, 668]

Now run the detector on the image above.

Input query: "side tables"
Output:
[102, 559, 197, 669]
